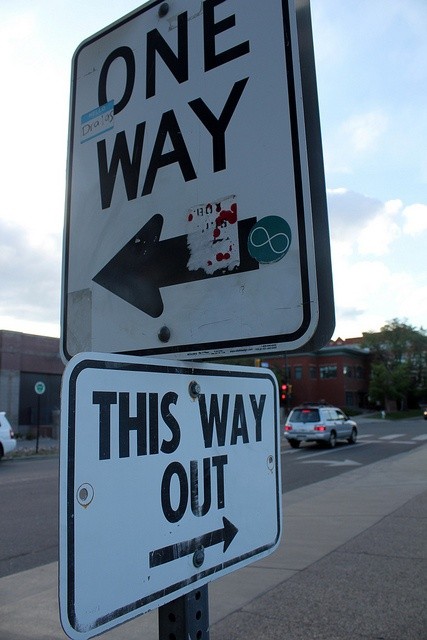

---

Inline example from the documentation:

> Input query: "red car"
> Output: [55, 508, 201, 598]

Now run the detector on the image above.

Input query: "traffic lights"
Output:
[281, 383, 286, 401]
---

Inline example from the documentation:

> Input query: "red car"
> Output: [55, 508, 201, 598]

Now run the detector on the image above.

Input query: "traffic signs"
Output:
[58, 351, 283, 640]
[59, 0, 336, 358]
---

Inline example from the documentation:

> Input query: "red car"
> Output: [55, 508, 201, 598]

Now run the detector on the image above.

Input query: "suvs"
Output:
[0, 412, 16, 456]
[284, 406, 358, 448]
